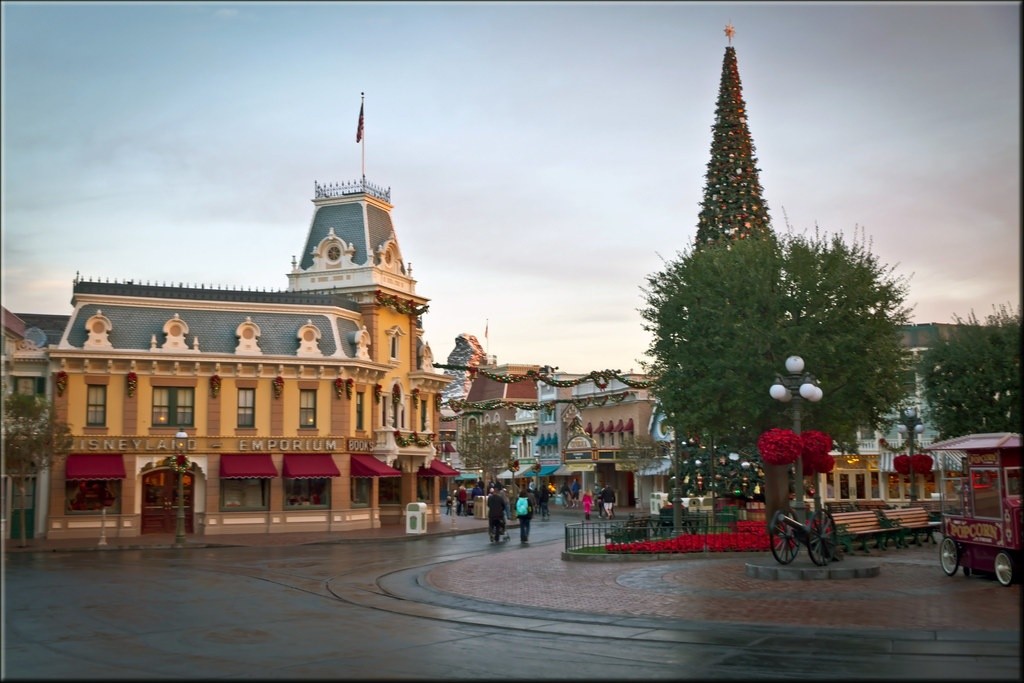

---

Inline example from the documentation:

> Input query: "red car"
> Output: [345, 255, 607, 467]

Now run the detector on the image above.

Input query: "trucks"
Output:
[922, 433, 1022, 586]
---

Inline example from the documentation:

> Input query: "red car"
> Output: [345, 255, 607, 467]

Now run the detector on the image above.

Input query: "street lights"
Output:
[175, 427, 186, 543]
[770, 355, 824, 551]
[509, 441, 517, 520]
[534, 450, 540, 489]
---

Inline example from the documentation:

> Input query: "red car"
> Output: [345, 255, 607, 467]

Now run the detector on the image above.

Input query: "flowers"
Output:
[272, 376, 285, 399]
[394, 430, 410, 446]
[210, 375, 222, 397]
[412, 431, 437, 447]
[334, 377, 343, 400]
[411, 388, 419, 409]
[170, 452, 195, 473]
[375, 383, 382, 404]
[435, 393, 442, 411]
[55, 371, 68, 396]
[392, 384, 401, 403]
[127, 371, 138, 397]
[346, 377, 354, 400]
[509, 459, 520, 472]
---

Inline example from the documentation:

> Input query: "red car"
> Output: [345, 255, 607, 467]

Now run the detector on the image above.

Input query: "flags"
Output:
[355, 101, 365, 144]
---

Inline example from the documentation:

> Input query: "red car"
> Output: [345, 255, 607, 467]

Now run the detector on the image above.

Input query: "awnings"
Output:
[280, 453, 342, 479]
[416, 458, 459, 477]
[350, 454, 401, 479]
[219, 453, 277, 479]
[65, 452, 126, 480]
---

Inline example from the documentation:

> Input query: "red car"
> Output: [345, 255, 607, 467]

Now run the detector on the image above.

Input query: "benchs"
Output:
[822, 506, 903, 556]
[872, 504, 943, 550]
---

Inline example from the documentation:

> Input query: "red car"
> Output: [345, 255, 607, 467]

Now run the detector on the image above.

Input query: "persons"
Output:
[583, 488, 593, 519]
[597, 487, 604, 518]
[487, 487, 505, 542]
[603, 484, 615, 518]
[436, 473, 582, 517]
[516, 490, 534, 543]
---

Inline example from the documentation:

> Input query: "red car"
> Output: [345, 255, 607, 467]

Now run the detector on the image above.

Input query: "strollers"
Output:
[492, 511, 511, 542]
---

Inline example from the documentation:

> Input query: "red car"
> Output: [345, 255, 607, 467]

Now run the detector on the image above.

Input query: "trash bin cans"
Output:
[405, 502, 428, 534]
[474, 495, 491, 519]
[650, 492, 669, 515]
